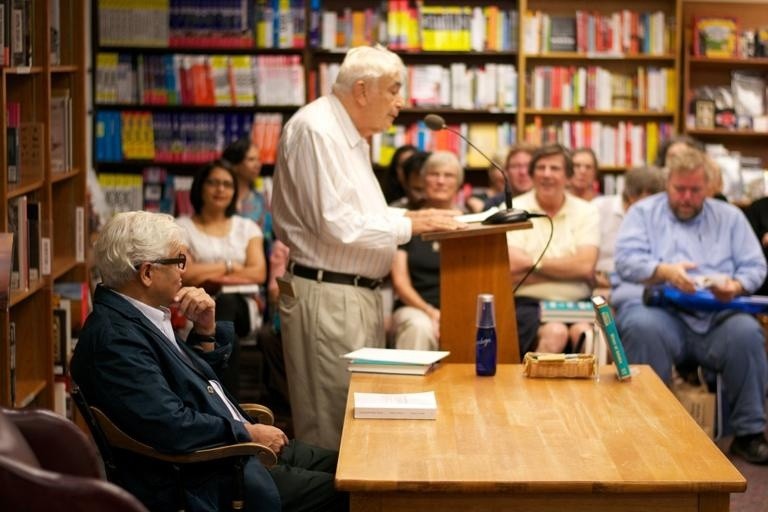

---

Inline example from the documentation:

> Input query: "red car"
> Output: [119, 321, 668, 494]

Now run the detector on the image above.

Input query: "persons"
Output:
[73, 212, 348, 512]
[705, 160, 768, 328]
[271, 43, 463, 452]
[612, 148, 768, 465]
[176, 143, 289, 419]
[388, 144, 668, 364]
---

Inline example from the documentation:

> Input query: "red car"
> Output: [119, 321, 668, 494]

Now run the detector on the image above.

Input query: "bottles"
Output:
[476, 295, 496, 376]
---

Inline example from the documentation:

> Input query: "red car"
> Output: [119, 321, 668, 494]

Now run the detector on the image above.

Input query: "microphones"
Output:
[425, 113, 529, 224]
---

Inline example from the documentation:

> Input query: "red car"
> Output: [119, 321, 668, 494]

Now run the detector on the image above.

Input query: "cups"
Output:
[476, 294, 496, 329]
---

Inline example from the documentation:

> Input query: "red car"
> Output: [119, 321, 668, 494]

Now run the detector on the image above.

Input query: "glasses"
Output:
[134, 253, 186, 271]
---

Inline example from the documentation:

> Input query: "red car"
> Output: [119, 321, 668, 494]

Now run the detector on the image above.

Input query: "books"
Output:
[367, 121, 517, 167]
[0, 2, 91, 416]
[524, 115, 673, 167]
[96, 54, 305, 105]
[526, 8, 767, 59]
[308, 1, 517, 53]
[310, 64, 516, 110]
[95, 112, 291, 164]
[353, 393, 439, 421]
[540, 300, 596, 324]
[99, 167, 273, 222]
[95, 1, 306, 49]
[523, 64, 766, 133]
[705, 145, 765, 205]
[342, 349, 451, 376]
[592, 295, 632, 381]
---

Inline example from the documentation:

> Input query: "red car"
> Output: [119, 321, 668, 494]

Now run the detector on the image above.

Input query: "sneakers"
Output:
[729, 433, 768, 464]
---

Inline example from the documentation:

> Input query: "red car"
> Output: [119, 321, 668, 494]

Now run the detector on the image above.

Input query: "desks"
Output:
[333, 356, 747, 512]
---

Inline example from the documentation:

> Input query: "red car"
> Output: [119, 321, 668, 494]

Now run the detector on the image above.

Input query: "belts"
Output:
[285, 259, 382, 289]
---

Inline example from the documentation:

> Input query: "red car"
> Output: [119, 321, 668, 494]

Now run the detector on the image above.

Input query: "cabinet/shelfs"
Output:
[95, 0, 683, 238]
[683, 0, 768, 214]
[0, 0, 95, 417]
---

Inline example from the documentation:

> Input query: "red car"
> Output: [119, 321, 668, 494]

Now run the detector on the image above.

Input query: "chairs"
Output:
[0, 407, 142, 512]
[71, 381, 278, 512]
